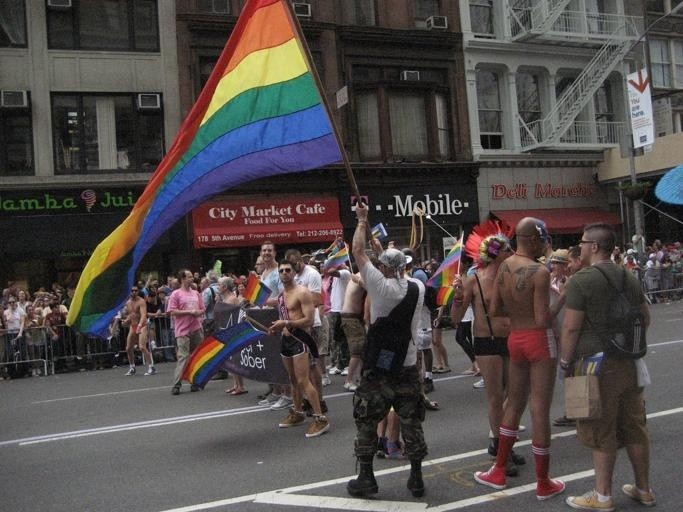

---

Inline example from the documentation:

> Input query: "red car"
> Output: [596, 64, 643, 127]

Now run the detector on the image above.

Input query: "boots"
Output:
[407, 469, 425, 498]
[346, 463, 380, 495]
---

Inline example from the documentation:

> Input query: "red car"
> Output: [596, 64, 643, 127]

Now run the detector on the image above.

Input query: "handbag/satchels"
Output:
[561, 371, 604, 421]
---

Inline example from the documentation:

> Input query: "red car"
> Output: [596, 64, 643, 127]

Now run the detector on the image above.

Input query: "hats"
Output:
[376, 246, 418, 271]
[549, 245, 571, 266]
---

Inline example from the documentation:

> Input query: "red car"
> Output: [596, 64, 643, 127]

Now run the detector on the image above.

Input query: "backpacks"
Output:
[411, 264, 441, 315]
[582, 259, 648, 360]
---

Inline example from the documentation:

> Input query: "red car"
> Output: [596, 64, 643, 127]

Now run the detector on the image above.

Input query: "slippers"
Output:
[224, 386, 249, 397]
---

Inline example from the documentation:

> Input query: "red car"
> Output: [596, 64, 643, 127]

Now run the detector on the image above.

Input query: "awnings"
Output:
[193, 194, 343, 248]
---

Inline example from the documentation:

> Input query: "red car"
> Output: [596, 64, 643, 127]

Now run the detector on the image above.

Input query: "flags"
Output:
[65, 0, 344, 342]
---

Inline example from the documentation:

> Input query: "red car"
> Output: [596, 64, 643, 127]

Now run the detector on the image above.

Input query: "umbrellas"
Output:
[654, 165, 683, 207]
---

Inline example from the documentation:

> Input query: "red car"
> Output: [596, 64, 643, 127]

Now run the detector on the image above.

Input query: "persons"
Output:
[1, 272, 183, 380]
[610, 228, 683, 305]
[166, 241, 355, 436]
[383, 217, 657, 510]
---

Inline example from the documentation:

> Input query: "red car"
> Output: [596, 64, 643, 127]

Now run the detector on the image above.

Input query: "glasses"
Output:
[277, 268, 292, 274]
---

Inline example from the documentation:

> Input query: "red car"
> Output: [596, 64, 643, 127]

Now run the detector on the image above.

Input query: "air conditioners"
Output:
[292, 3, 312, 18]
[137, 93, 161, 112]
[399, 70, 420, 81]
[0, 89, 28, 108]
[47, 0, 72, 7]
[425, 15, 448, 31]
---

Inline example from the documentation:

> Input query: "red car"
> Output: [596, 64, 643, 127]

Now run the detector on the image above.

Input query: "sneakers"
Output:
[257, 364, 361, 439]
[1, 362, 120, 383]
[487, 439, 527, 477]
[210, 369, 227, 380]
[550, 415, 579, 427]
[461, 367, 480, 377]
[125, 367, 136, 376]
[422, 374, 434, 395]
[142, 365, 159, 376]
[473, 465, 507, 490]
[170, 386, 180, 395]
[376, 434, 404, 459]
[472, 378, 486, 388]
[622, 483, 657, 507]
[432, 365, 451, 373]
[564, 493, 618, 512]
[535, 476, 566, 500]
